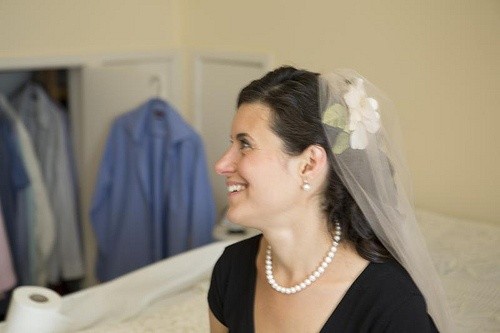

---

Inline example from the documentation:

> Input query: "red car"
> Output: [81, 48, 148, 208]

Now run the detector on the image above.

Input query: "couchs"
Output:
[58, 205, 500, 333]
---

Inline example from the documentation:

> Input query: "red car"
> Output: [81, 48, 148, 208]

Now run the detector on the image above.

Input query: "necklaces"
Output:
[265, 214, 342, 293]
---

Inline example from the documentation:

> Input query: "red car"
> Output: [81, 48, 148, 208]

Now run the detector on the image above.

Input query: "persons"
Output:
[207, 64, 457, 333]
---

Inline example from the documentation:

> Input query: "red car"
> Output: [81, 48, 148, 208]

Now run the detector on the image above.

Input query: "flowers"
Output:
[321, 78, 382, 153]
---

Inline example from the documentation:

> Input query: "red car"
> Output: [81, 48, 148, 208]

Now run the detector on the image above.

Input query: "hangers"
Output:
[113, 78, 200, 146]
